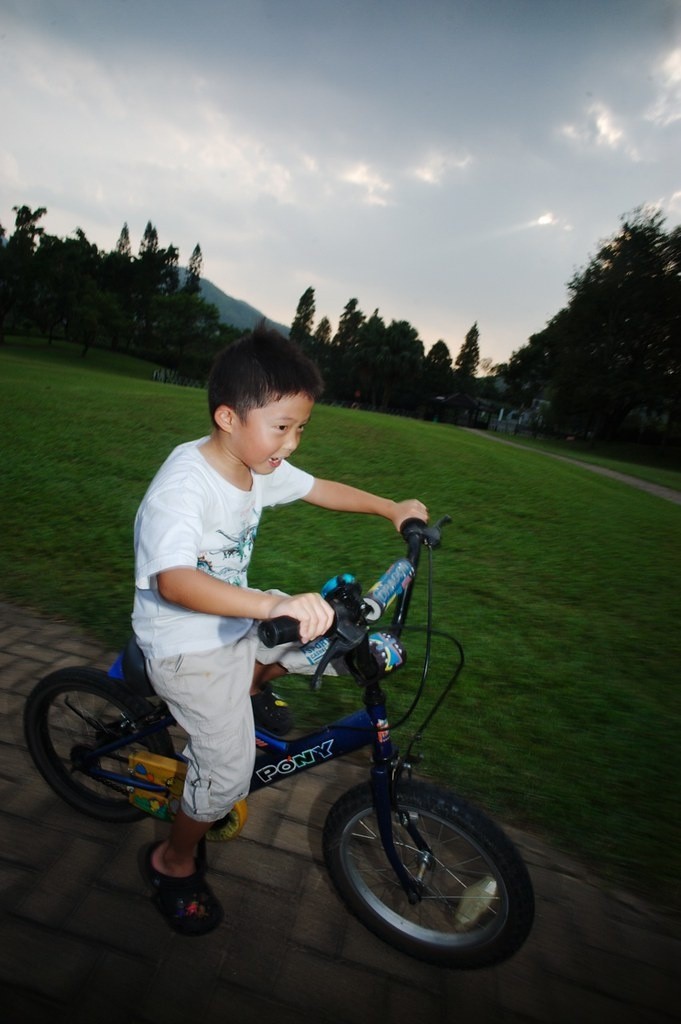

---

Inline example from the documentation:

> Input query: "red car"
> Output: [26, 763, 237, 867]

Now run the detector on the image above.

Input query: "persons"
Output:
[132, 331, 429, 930]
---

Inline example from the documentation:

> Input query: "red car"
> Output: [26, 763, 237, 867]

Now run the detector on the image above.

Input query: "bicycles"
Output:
[20, 509, 540, 972]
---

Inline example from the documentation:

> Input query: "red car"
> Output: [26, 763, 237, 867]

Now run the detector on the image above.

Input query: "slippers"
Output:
[137, 839, 226, 937]
[251, 682, 296, 735]
